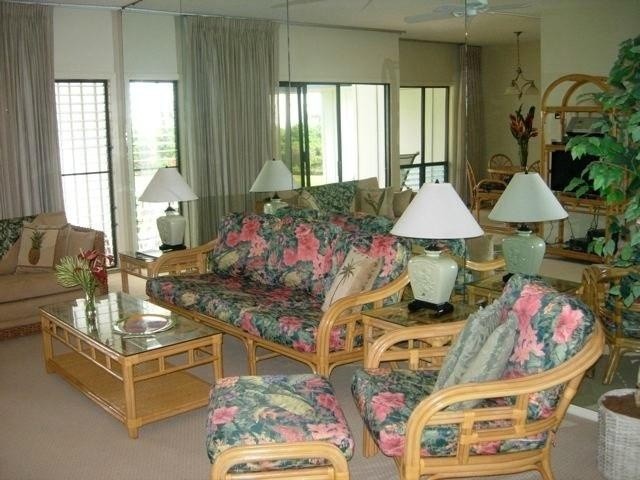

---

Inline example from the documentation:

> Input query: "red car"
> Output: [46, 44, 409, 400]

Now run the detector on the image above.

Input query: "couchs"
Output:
[298, 176, 418, 216]
[145, 213, 426, 377]
[0, 215, 109, 341]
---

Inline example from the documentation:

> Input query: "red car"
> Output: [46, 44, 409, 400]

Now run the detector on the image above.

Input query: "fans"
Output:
[404, 0, 546, 24]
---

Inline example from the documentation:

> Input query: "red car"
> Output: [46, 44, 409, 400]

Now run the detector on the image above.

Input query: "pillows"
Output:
[431, 300, 503, 394]
[298, 190, 321, 211]
[450, 313, 518, 414]
[14, 220, 72, 274]
[358, 187, 412, 216]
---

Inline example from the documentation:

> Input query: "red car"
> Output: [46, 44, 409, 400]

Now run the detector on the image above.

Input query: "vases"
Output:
[85, 290, 96, 324]
[518, 143, 529, 171]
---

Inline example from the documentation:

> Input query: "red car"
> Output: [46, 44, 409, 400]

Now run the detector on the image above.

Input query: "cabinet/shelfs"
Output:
[541, 73, 626, 265]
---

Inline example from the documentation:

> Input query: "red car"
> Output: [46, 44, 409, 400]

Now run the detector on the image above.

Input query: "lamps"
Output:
[249, 160, 301, 217]
[504, 32, 539, 96]
[488, 173, 569, 280]
[388, 182, 485, 317]
[138, 167, 200, 252]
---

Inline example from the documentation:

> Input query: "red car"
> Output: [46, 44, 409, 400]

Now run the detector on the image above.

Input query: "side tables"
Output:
[118, 254, 198, 294]
[359, 300, 481, 367]
[465, 273, 584, 303]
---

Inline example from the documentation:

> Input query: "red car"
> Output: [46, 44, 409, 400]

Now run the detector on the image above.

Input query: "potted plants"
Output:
[564, 36, 639, 480]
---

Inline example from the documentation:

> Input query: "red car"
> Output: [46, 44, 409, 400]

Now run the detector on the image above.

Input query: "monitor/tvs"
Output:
[549, 140, 608, 200]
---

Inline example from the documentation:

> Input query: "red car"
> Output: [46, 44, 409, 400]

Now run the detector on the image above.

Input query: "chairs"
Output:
[352, 274, 606, 480]
[464, 153, 540, 223]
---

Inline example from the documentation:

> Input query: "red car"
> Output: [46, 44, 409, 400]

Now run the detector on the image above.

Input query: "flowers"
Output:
[507, 104, 537, 140]
[54, 248, 116, 296]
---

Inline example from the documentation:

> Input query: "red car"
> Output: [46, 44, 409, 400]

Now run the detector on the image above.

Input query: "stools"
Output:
[207, 372, 356, 479]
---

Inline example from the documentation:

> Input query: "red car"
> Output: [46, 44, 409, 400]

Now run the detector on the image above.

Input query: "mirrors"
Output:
[120, 0, 640, 413]
